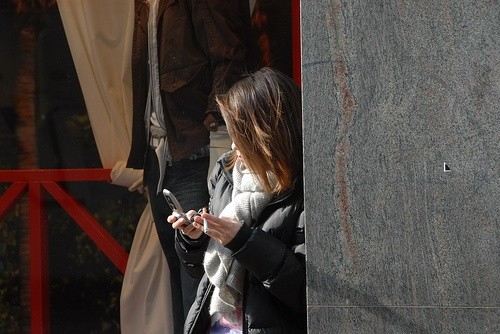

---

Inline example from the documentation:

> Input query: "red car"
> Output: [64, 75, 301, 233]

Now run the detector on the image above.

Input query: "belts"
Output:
[148, 136, 161, 148]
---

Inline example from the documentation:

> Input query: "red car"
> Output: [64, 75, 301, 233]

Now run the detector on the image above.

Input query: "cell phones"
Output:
[162, 188, 191, 229]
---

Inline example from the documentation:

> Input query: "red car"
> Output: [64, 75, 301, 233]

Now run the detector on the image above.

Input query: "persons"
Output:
[167, 66, 306, 334]
[126, 1, 247, 334]
